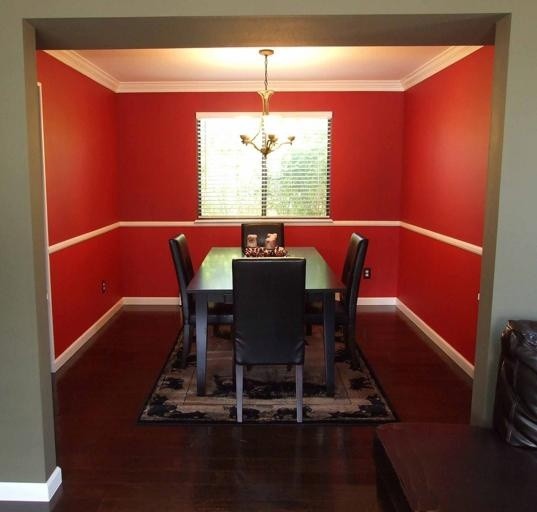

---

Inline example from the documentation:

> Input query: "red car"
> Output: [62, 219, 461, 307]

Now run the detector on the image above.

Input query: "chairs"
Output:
[232, 258, 306, 423]
[167, 232, 235, 367]
[240, 222, 284, 249]
[306, 232, 370, 370]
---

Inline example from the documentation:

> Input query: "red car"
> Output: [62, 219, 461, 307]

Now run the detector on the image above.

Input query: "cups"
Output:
[246, 234, 258, 248]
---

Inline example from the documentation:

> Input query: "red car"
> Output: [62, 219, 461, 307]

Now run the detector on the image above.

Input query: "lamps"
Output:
[239, 49, 295, 159]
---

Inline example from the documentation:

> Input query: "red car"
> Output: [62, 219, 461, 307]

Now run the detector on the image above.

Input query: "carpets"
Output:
[137, 323, 400, 424]
